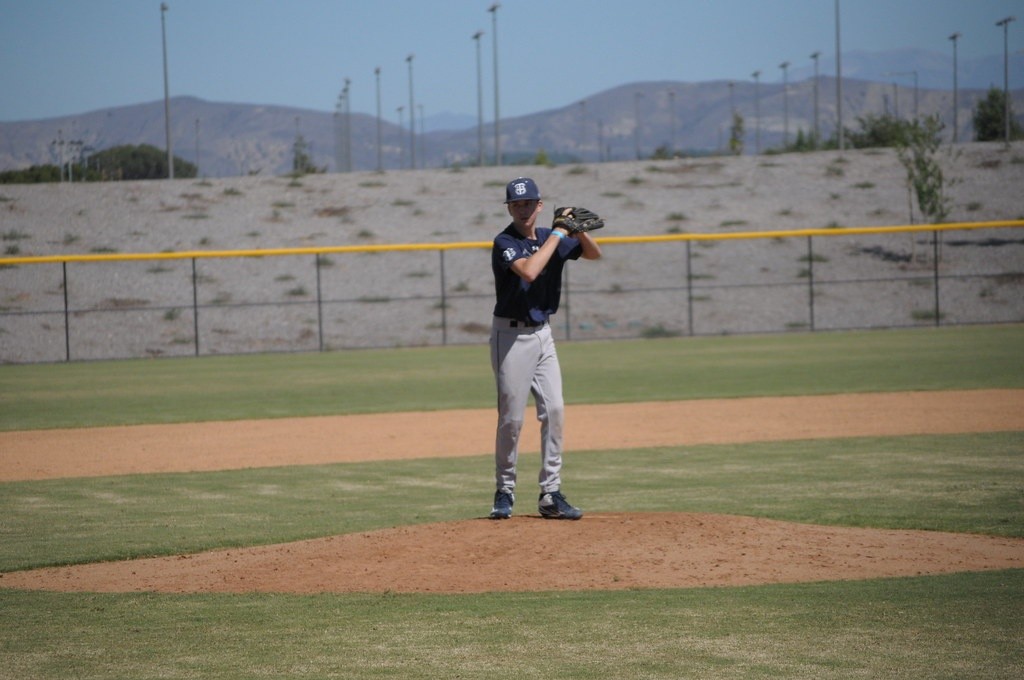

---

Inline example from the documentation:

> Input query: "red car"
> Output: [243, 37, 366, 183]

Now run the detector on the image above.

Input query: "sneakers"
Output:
[538, 490, 582, 519]
[490, 489, 515, 518]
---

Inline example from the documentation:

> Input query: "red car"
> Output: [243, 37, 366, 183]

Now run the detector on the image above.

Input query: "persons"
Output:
[490, 177, 601, 519]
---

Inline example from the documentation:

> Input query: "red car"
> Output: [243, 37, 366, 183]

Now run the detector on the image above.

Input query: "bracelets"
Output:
[551, 230, 565, 240]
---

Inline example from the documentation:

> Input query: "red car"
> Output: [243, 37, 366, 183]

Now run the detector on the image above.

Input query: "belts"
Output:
[510, 319, 548, 327]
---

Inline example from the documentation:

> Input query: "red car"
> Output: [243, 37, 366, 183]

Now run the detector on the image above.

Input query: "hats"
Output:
[504, 176, 540, 202]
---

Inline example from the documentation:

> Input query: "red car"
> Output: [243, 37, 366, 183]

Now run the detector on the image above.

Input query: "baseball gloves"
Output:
[552, 205, 606, 234]
